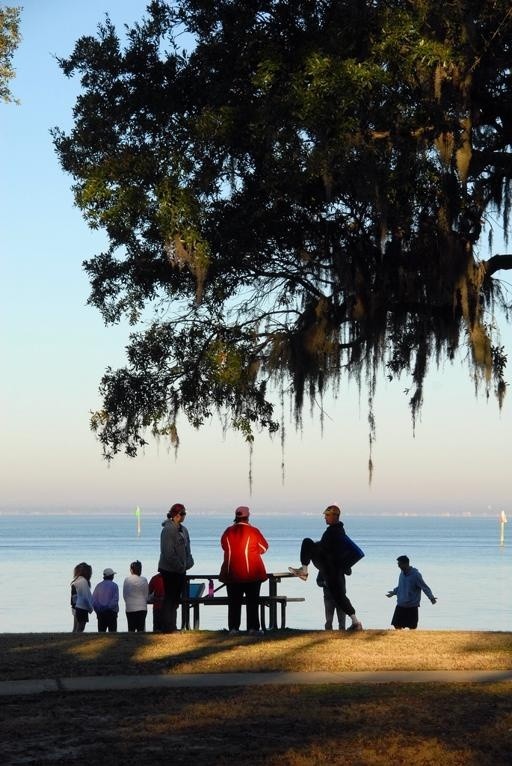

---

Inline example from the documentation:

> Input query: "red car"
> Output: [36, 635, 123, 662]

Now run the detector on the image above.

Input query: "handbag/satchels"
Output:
[342, 535, 364, 570]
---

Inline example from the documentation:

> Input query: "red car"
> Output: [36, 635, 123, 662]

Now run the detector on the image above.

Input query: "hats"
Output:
[323, 505, 340, 515]
[104, 568, 115, 576]
[236, 507, 249, 517]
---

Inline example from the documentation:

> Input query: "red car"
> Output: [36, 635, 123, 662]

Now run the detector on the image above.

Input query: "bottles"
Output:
[209, 580, 214, 598]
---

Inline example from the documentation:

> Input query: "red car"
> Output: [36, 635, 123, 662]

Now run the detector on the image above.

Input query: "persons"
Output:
[123, 561, 155, 634]
[69, 562, 95, 632]
[91, 566, 120, 632]
[313, 568, 352, 630]
[217, 506, 269, 638]
[383, 555, 438, 629]
[285, 505, 365, 632]
[146, 569, 166, 633]
[159, 503, 196, 633]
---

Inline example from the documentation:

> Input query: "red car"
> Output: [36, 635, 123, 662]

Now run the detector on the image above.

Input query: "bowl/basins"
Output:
[190, 584, 206, 598]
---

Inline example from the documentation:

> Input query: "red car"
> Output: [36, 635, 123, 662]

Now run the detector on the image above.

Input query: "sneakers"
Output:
[289, 567, 308, 581]
[249, 629, 264, 635]
[347, 622, 362, 631]
[229, 629, 238, 636]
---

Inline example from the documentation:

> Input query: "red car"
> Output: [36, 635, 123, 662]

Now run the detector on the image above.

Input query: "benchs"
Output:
[179, 572, 306, 631]
